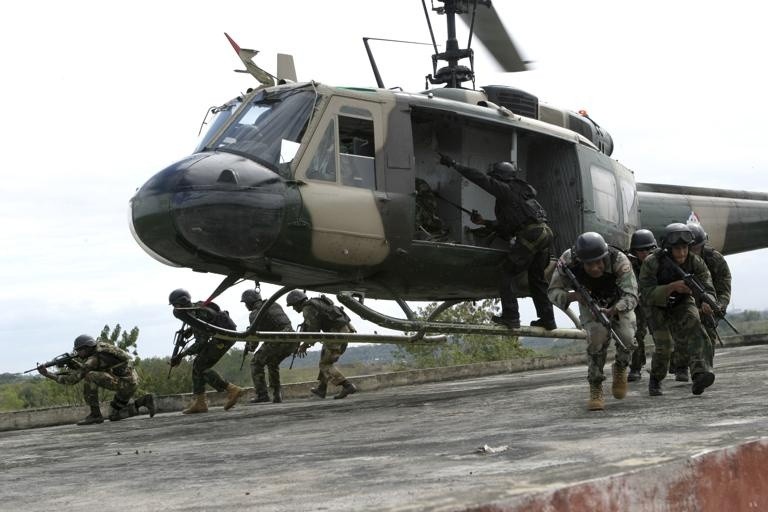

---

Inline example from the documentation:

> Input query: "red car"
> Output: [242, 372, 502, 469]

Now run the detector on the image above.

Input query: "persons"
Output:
[637, 222, 720, 397]
[435, 149, 557, 331]
[284, 290, 360, 399]
[241, 290, 298, 404]
[170, 287, 245, 415]
[546, 234, 640, 412]
[415, 176, 443, 231]
[39, 334, 156, 426]
[667, 223, 732, 382]
[620, 227, 663, 381]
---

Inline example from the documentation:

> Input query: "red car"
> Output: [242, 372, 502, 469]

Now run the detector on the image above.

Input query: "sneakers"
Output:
[310, 388, 325, 398]
[78, 415, 104, 425]
[530, 317, 556, 330]
[627, 366, 715, 395]
[492, 315, 520, 328]
[334, 384, 357, 399]
[143, 394, 155, 417]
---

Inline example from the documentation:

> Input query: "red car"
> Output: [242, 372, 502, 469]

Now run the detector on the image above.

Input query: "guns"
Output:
[290, 325, 304, 369]
[23, 353, 79, 373]
[658, 251, 734, 345]
[168, 322, 187, 378]
[558, 257, 629, 351]
[240, 340, 255, 368]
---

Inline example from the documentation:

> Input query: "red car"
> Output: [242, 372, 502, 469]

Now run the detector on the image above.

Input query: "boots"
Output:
[183, 393, 208, 414]
[250, 390, 269, 403]
[610, 364, 627, 399]
[224, 383, 242, 410]
[589, 382, 604, 411]
[273, 387, 282, 402]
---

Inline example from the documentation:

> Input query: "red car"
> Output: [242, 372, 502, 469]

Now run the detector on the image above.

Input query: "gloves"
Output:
[183, 329, 192, 339]
[438, 152, 452, 168]
[171, 353, 182, 367]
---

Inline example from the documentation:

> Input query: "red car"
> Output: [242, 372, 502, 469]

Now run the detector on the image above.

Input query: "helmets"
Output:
[169, 289, 191, 306]
[240, 290, 261, 305]
[572, 222, 709, 263]
[74, 335, 97, 350]
[287, 291, 307, 306]
[493, 161, 517, 181]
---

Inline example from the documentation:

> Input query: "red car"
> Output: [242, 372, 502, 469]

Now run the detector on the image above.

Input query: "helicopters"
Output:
[129, 0, 768, 344]
